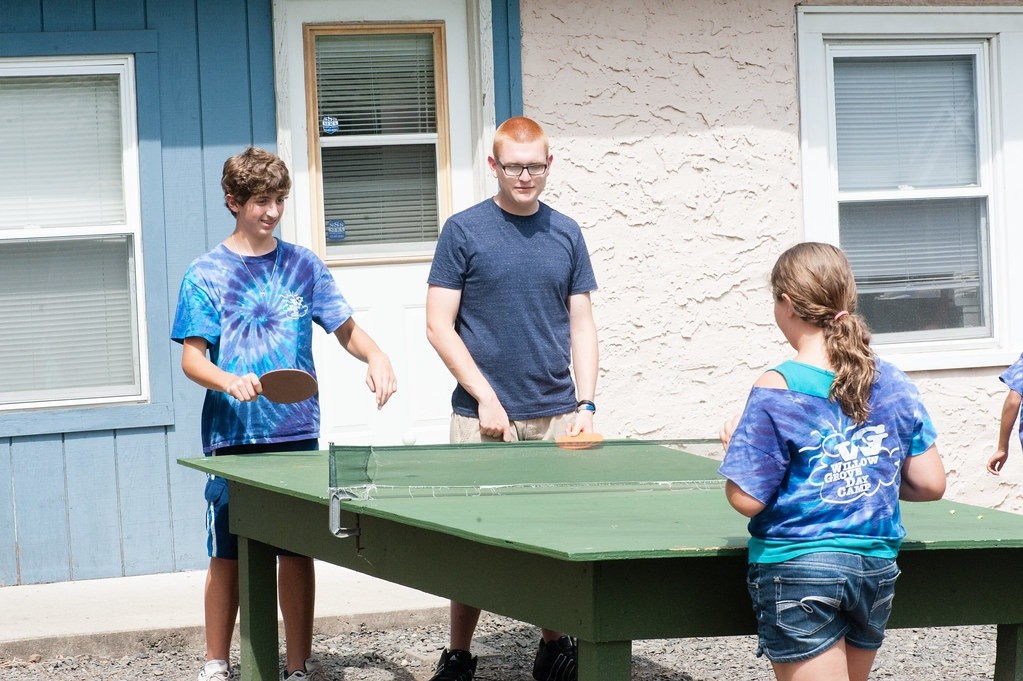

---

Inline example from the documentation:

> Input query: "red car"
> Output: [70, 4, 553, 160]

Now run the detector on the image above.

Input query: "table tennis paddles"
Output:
[556, 429, 604, 452]
[252, 370, 317, 404]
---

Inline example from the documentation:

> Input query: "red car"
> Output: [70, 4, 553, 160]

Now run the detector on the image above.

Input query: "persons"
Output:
[985, 351, 1022, 476]
[168, 147, 398, 681]
[420, 116, 603, 680]
[717, 242, 946, 681]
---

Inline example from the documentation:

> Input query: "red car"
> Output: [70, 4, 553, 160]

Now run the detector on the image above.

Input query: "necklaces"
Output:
[230, 234, 278, 297]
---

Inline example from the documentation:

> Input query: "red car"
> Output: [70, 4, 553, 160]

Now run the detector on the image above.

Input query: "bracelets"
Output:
[575, 399, 596, 416]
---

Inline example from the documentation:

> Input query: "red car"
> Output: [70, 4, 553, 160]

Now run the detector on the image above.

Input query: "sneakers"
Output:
[198, 659, 228, 681]
[533, 637, 578, 681]
[281, 669, 310, 681]
[427, 649, 478, 681]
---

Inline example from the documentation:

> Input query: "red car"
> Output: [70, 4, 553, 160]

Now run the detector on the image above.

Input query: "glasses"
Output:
[493, 155, 547, 176]
